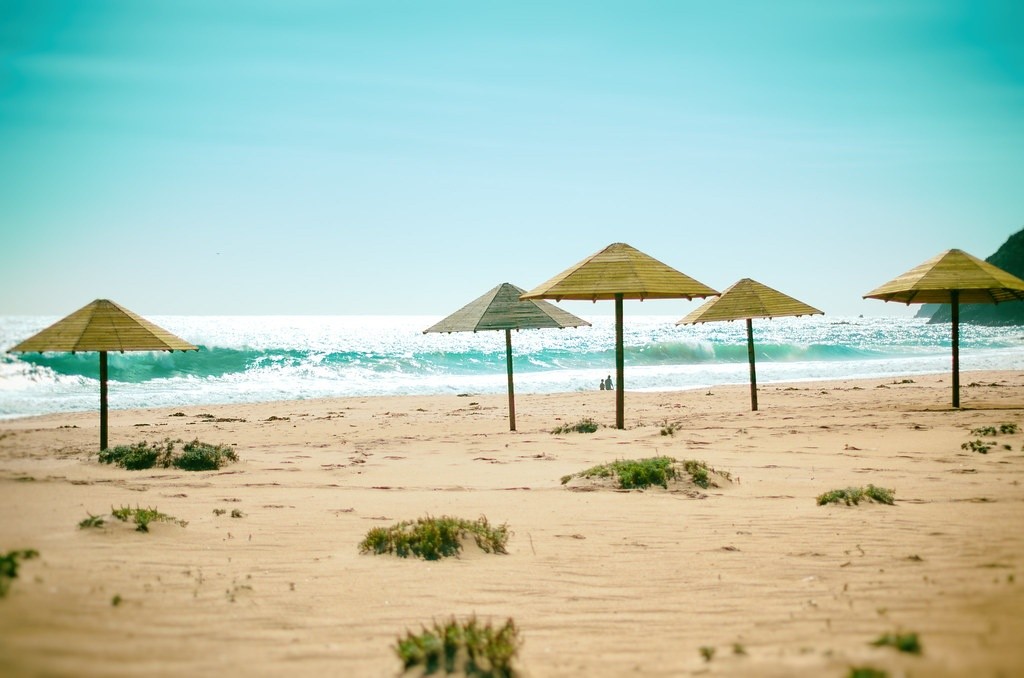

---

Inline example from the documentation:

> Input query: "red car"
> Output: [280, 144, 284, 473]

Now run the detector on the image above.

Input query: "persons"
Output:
[600, 375, 614, 390]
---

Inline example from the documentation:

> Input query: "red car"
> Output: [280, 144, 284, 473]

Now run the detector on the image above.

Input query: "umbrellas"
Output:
[422, 283, 592, 430]
[675, 278, 825, 412]
[519, 242, 721, 432]
[6, 299, 199, 460]
[862, 248, 1024, 408]
